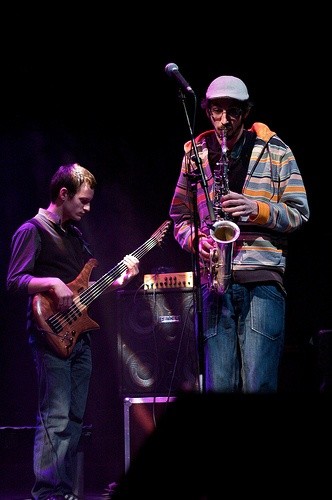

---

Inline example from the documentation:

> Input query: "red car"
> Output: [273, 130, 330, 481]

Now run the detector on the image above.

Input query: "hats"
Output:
[206, 75, 250, 101]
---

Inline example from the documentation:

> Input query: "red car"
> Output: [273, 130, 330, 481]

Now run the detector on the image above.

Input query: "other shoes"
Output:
[46, 492, 80, 500]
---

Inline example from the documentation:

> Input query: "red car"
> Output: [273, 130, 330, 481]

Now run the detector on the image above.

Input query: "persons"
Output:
[169, 75, 311, 391]
[4, 163, 141, 500]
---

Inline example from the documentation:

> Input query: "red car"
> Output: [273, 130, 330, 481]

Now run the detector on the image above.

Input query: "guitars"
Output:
[31, 218, 172, 358]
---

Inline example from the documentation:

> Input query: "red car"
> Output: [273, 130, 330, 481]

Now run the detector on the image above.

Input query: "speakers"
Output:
[116, 287, 198, 397]
[106, 390, 332, 500]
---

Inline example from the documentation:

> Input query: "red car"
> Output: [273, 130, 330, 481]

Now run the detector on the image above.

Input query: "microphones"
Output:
[165, 62, 196, 95]
[319, 330, 332, 335]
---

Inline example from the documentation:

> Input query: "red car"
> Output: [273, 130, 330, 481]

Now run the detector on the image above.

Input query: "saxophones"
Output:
[207, 126, 240, 297]
